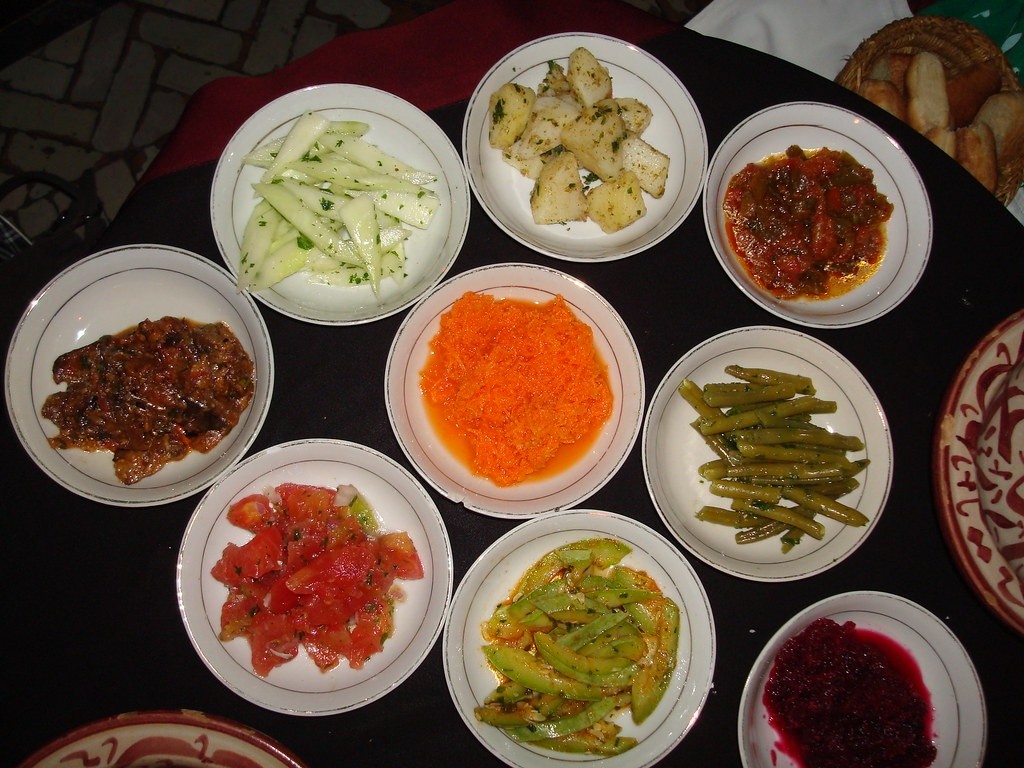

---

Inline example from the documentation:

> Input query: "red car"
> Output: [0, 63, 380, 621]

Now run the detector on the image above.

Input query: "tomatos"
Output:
[209, 483, 426, 678]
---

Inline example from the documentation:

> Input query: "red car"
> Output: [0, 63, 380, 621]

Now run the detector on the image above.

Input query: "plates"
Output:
[440, 508, 717, 768]
[175, 436, 454, 717]
[701, 100, 934, 330]
[642, 326, 894, 583]
[4, 243, 274, 508]
[383, 262, 646, 520]
[209, 82, 471, 327]
[930, 307, 1024, 638]
[460, 31, 709, 263]
[20, 707, 307, 768]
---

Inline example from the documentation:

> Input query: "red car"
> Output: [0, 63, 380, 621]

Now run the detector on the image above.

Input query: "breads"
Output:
[856, 50, 1023, 198]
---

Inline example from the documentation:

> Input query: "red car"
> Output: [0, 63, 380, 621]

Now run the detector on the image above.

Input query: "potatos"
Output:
[487, 46, 670, 233]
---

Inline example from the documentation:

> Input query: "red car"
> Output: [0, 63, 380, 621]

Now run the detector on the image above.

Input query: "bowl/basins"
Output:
[737, 589, 988, 768]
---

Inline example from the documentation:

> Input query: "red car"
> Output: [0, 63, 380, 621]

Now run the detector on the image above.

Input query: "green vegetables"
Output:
[242, 126, 427, 286]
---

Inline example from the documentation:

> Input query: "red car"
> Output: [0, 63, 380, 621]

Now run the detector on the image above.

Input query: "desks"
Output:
[0, 0, 1024, 768]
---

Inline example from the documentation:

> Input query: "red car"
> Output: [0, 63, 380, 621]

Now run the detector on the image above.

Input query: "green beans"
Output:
[679, 366, 869, 553]
